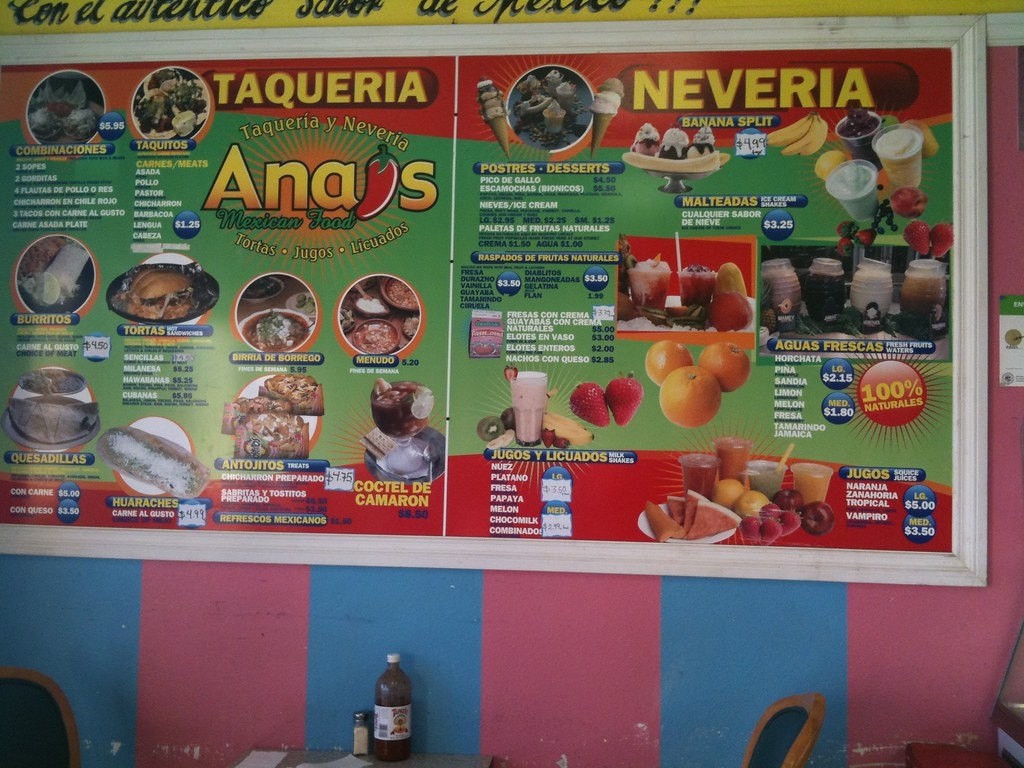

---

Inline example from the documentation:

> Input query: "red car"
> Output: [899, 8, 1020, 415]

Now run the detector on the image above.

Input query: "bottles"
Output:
[353, 712, 369, 757]
[373, 653, 412, 761]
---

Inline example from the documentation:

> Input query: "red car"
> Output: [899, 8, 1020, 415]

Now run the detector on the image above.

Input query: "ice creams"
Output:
[477, 74, 625, 160]
[630, 122, 716, 159]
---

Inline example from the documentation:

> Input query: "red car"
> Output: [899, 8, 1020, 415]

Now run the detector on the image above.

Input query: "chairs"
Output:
[741, 691, 825, 768]
[1, 668, 81, 768]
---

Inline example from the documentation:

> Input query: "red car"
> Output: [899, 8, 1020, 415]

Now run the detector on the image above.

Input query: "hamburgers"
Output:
[127, 267, 199, 321]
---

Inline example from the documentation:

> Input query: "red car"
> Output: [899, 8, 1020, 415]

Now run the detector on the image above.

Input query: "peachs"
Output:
[891, 186, 927, 218]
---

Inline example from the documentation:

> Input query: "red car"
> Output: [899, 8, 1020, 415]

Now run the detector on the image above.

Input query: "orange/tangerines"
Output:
[712, 479, 773, 526]
[644, 339, 750, 428]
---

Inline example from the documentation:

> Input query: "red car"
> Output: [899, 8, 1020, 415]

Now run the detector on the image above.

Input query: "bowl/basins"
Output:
[352, 295, 391, 317]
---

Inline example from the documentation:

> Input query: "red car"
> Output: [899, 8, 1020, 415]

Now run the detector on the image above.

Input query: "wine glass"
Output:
[370, 381, 432, 474]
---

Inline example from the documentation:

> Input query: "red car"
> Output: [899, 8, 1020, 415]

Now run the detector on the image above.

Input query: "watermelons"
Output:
[667, 489, 742, 540]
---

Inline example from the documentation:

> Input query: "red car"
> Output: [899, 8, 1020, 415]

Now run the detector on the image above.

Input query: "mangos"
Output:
[713, 262, 747, 298]
[903, 120, 939, 159]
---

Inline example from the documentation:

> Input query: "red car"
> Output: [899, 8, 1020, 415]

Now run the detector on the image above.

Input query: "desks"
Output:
[226, 744, 496, 768]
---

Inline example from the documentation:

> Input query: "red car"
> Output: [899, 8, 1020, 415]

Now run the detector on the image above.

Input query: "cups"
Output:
[744, 459, 788, 501]
[834, 111, 883, 172]
[677, 272, 717, 308]
[678, 453, 721, 502]
[627, 269, 672, 310]
[790, 462, 834, 505]
[825, 160, 880, 222]
[712, 437, 753, 485]
[871, 123, 924, 188]
[510, 372, 548, 446]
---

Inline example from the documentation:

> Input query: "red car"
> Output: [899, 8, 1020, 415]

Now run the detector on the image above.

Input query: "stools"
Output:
[905, 739, 1000, 768]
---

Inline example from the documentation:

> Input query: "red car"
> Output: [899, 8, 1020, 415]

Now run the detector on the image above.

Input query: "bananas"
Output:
[542, 412, 594, 446]
[765, 112, 828, 156]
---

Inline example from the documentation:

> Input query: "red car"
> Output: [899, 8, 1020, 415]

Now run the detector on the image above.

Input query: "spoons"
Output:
[355, 284, 372, 302]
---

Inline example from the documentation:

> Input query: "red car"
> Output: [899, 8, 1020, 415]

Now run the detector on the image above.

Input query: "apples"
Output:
[773, 489, 834, 534]
[880, 115, 898, 128]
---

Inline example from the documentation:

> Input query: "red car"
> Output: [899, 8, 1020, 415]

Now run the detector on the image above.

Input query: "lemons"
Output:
[815, 151, 848, 181]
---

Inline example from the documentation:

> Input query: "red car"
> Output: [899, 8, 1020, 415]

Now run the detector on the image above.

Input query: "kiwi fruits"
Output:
[477, 407, 514, 442]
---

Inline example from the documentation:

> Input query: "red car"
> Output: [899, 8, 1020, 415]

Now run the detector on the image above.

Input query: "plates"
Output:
[238, 308, 314, 354]
[2, 395, 100, 448]
[240, 277, 285, 304]
[400, 314, 416, 340]
[118, 416, 192, 496]
[637, 503, 736, 544]
[340, 307, 356, 333]
[380, 278, 419, 311]
[364, 425, 446, 485]
[352, 319, 399, 355]
[285, 292, 316, 317]
[239, 373, 318, 441]
[107, 263, 220, 323]
[505, 65, 595, 153]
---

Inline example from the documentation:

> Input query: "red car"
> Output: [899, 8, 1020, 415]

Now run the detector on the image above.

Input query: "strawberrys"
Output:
[903, 221, 954, 256]
[835, 222, 877, 256]
[570, 371, 643, 427]
[543, 428, 570, 448]
[739, 504, 801, 546]
